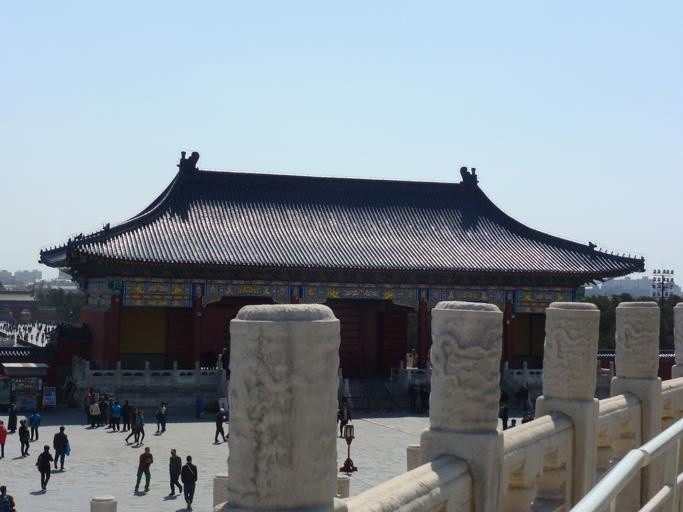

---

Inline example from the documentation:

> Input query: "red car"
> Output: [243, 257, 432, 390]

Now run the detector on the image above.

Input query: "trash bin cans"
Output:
[408, 385, 429, 414]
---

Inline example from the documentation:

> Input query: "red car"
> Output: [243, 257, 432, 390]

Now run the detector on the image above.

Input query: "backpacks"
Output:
[0, 494, 15, 511]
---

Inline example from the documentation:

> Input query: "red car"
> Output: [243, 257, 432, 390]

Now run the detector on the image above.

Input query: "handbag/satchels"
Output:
[65, 441, 73, 456]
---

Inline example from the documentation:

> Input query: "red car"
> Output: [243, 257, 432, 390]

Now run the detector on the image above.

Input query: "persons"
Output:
[0, 389, 228, 512]
[499, 385, 533, 429]
[337, 404, 352, 438]
[407, 380, 429, 415]
